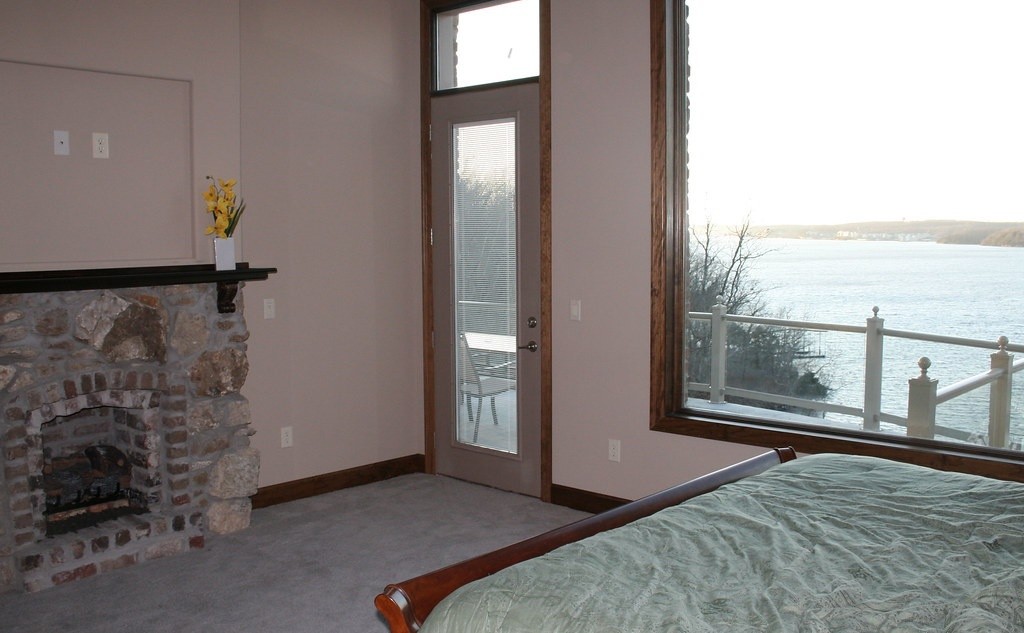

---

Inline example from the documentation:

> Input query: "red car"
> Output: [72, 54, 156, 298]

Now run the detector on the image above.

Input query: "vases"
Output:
[213, 236, 236, 273]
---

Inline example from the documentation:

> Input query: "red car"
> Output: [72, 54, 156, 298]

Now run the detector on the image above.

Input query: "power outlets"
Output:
[53, 129, 70, 155]
[91, 132, 109, 160]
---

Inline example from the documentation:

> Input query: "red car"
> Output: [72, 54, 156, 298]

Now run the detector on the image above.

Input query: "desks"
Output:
[464, 333, 517, 372]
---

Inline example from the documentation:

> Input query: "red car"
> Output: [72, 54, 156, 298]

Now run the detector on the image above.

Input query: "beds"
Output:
[374, 447, 1024, 632]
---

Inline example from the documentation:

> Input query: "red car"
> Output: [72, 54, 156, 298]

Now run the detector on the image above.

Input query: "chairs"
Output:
[455, 329, 517, 444]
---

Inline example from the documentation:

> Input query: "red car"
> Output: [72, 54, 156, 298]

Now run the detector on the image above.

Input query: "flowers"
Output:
[198, 174, 246, 238]
